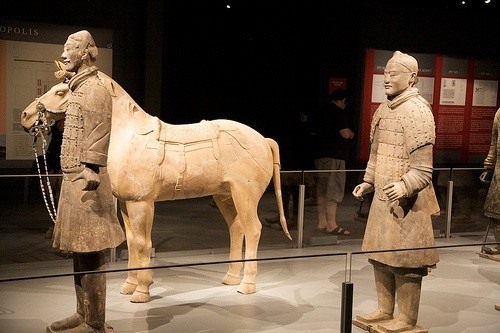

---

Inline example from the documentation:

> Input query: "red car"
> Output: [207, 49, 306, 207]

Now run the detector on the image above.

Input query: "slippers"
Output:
[318, 226, 351, 236]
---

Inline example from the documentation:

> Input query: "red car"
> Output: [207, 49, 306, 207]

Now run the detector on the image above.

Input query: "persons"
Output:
[314, 88, 355, 236]
[351, 49, 439, 332]
[479, 107, 500, 254]
[42, 117, 68, 172]
[265, 173, 301, 230]
[45, 30, 126, 333]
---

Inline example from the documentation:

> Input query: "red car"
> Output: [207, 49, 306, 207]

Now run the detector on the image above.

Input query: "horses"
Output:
[21, 60, 292, 303]
